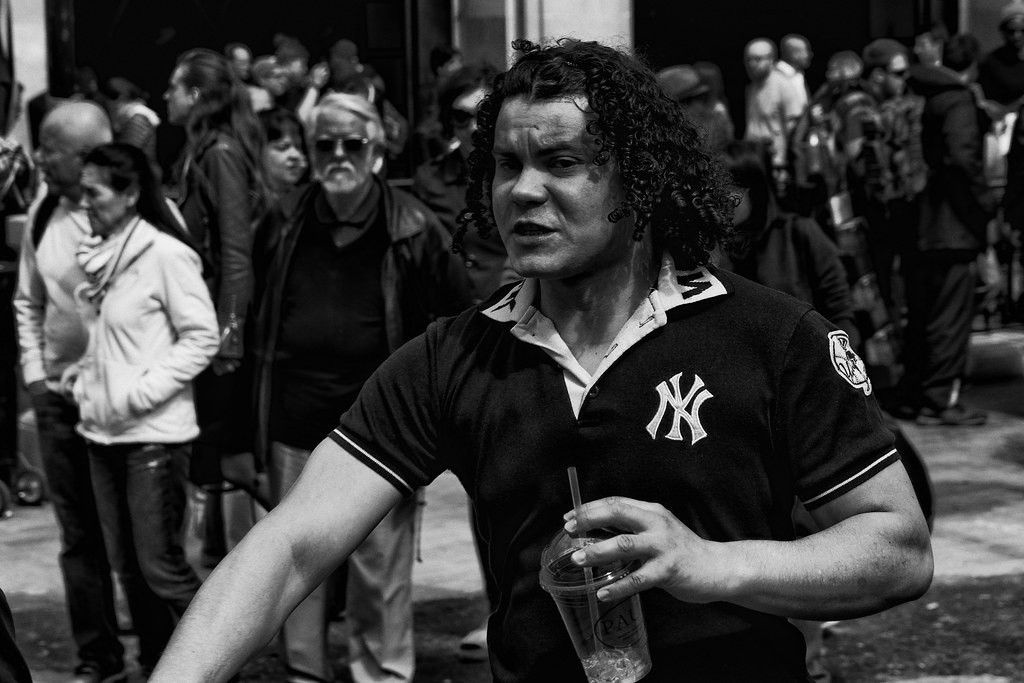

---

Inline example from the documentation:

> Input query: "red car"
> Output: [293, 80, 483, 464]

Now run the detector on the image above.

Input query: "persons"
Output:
[0, 25, 512, 683]
[144, 32, 939, 683]
[654, 0, 1023, 430]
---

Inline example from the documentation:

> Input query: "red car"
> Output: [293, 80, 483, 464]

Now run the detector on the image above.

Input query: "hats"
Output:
[659, 67, 709, 97]
[997, 1, 1024, 25]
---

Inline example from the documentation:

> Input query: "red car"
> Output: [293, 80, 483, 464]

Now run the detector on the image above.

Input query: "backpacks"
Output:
[860, 93, 925, 203]
[787, 95, 872, 231]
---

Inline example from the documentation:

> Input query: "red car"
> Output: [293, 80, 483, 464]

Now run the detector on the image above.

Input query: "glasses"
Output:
[456, 102, 486, 131]
[314, 134, 374, 153]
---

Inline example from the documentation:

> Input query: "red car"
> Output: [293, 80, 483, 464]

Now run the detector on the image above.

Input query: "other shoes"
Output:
[886, 394, 925, 421]
[919, 401, 987, 427]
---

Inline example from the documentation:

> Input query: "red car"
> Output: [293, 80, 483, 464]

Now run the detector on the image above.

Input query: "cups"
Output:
[538, 525, 653, 683]
